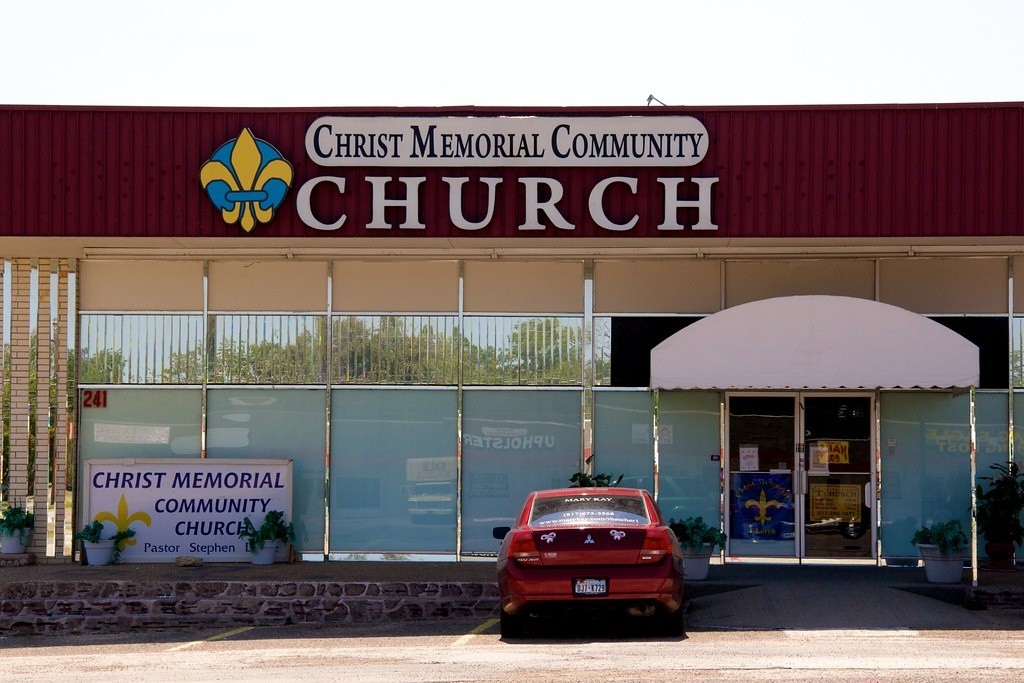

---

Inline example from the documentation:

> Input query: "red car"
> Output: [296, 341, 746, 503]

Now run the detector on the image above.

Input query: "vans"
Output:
[406, 481, 456, 523]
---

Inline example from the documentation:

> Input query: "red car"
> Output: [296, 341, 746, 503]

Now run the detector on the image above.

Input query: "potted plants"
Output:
[74, 520, 137, 567]
[669, 517, 729, 580]
[965, 461, 1024, 569]
[908, 519, 971, 583]
[238, 511, 299, 565]
[0, 503, 33, 554]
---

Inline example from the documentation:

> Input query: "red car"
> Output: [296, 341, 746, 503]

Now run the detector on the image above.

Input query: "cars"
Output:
[492, 485, 687, 640]
